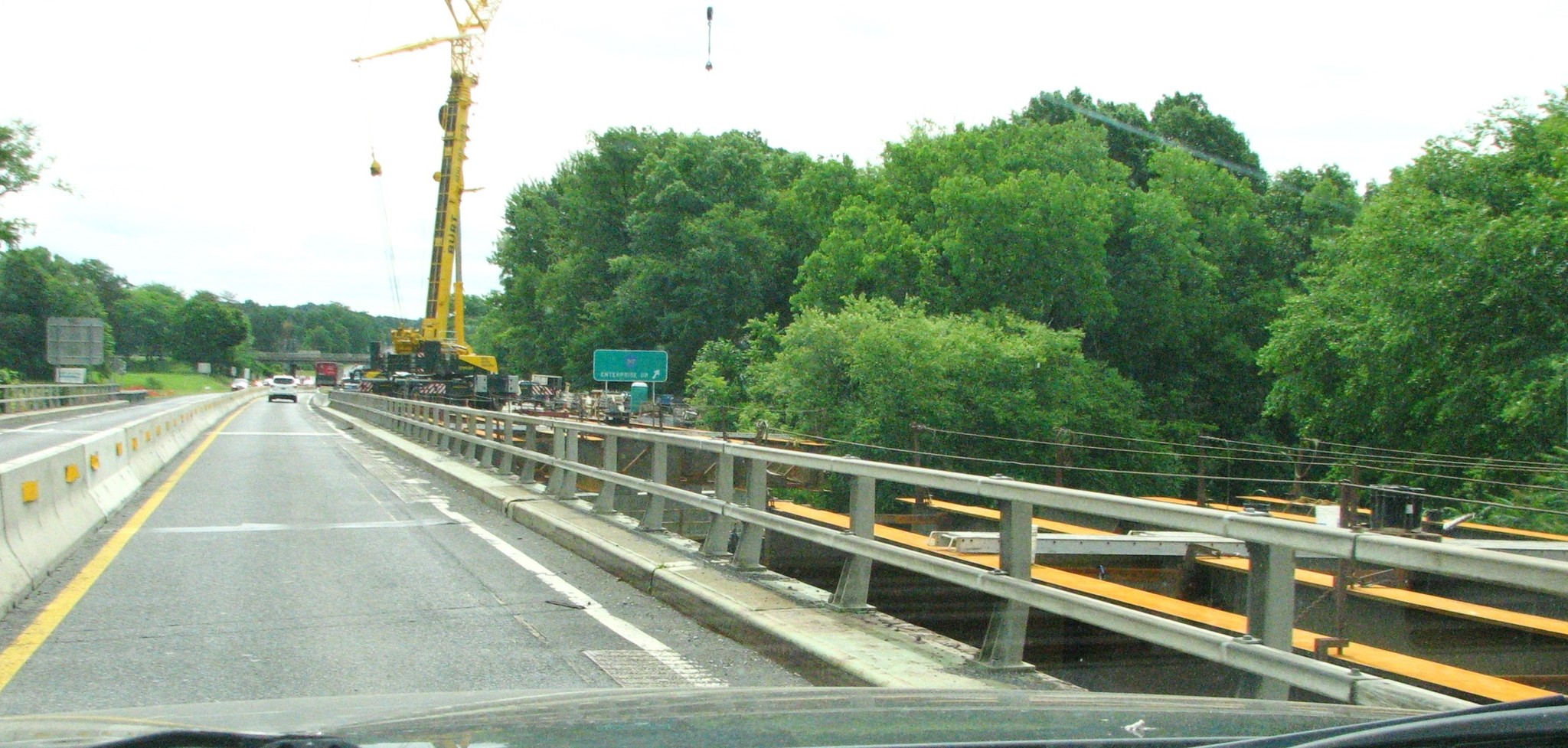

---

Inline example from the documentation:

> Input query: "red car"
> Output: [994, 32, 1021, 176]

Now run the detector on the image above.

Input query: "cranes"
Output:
[350, 0, 503, 376]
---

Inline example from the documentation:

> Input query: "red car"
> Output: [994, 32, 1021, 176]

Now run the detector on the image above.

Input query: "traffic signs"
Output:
[593, 350, 668, 382]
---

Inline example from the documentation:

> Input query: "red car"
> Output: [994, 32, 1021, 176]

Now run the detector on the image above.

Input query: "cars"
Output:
[269, 374, 297, 404]
[232, 379, 249, 391]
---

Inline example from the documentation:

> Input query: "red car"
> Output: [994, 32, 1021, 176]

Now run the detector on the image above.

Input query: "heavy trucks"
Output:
[340, 365, 519, 412]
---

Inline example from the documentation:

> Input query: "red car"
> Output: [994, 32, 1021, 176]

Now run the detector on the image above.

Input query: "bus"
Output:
[315, 360, 344, 387]
